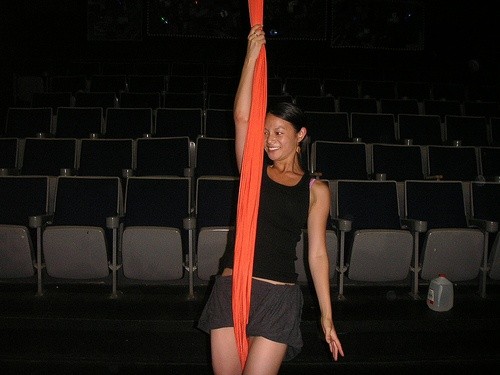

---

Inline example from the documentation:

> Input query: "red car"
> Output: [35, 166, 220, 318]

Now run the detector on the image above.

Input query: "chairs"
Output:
[0, 54, 500, 303]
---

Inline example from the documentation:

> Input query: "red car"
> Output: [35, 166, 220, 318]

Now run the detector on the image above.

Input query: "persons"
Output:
[464, 56, 480, 100]
[197, 25, 344, 375]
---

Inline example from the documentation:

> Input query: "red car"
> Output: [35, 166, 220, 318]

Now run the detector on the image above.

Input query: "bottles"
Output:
[427, 274, 454, 312]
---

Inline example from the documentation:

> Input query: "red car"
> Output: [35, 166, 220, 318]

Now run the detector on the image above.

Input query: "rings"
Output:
[254, 32, 258, 37]
